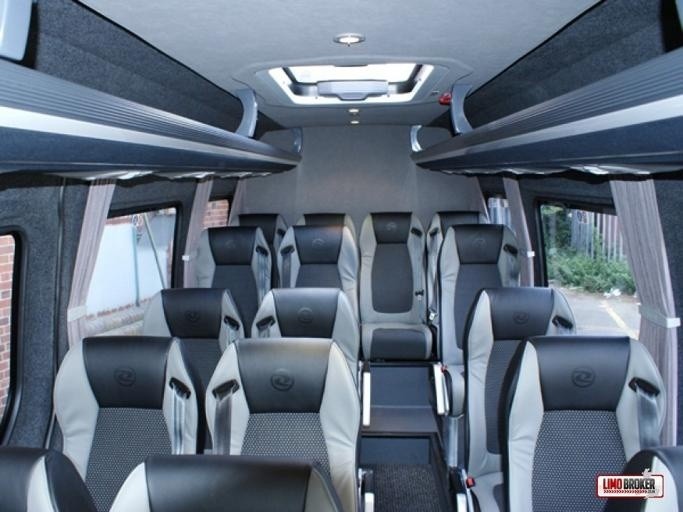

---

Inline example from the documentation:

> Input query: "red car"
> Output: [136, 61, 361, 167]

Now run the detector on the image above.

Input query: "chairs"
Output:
[0, 210, 683, 512]
